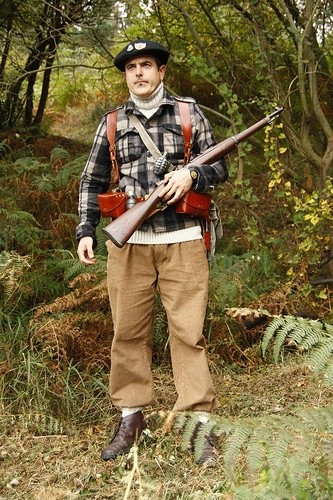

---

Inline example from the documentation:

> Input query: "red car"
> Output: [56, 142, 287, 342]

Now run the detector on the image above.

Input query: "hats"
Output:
[113, 39, 171, 72]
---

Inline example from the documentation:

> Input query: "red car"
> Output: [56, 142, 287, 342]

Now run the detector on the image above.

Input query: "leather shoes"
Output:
[100, 410, 147, 460]
[185, 419, 219, 469]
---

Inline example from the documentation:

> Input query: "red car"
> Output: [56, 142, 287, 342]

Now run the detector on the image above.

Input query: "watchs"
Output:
[190, 167, 198, 187]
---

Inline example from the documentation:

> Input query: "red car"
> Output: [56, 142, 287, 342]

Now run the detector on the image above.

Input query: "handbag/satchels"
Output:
[96, 192, 128, 218]
[174, 188, 212, 221]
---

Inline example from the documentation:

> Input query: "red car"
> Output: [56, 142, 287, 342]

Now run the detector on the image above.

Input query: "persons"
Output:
[75, 39, 229, 467]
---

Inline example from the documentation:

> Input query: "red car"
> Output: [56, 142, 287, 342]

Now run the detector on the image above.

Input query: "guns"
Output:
[103, 105, 287, 248]
